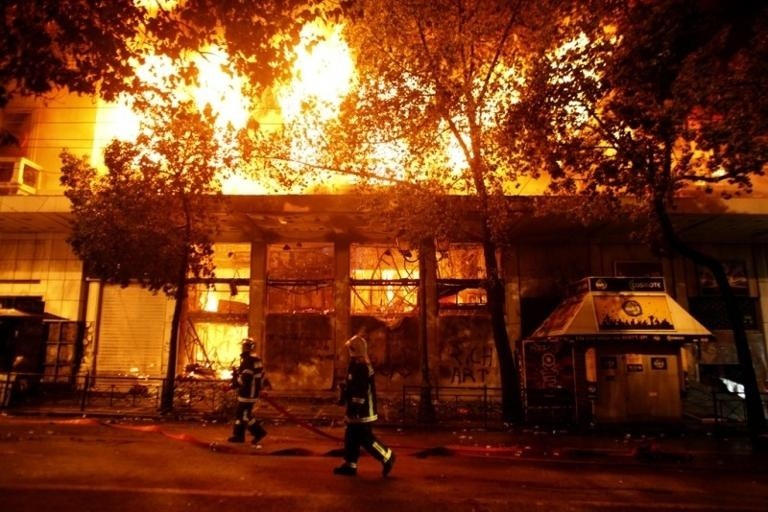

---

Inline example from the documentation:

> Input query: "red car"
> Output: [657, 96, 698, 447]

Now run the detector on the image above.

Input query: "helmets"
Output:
[239, 339, 255, 351]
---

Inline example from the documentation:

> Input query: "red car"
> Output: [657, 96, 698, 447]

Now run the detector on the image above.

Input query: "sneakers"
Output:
[382, 454, 395, 478]
[252, 433, 266, 445]
[229, 436, 244, 443]
[334, 464, 356, 475]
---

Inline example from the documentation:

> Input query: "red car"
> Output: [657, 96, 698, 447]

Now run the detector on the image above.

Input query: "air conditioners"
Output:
[0, 155, 43, 196]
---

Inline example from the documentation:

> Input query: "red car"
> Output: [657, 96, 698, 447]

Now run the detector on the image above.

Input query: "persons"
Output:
[332, 333, 396, 477]
[227, 339, 269, 444]
